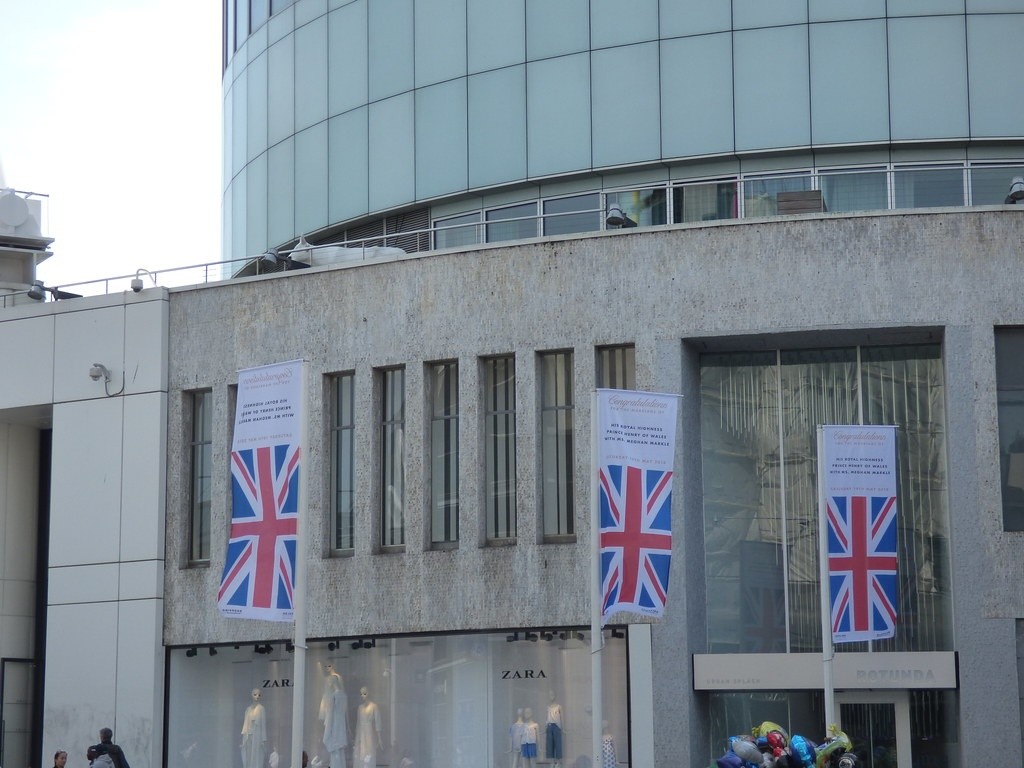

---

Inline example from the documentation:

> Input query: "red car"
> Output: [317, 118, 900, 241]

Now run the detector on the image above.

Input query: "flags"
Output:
[596, 388, 679, 620]
[215, 358, 303, 623]
[824, 425, 898, 643]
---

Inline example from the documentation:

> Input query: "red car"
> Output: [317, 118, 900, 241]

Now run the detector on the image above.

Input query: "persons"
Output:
[353, 686, 386, 768]
[603, 719, 622, 768]
[52, 750, 68, 768]
[504, 708, 541, 768]
[240, 688, 268, 768]
[318, 660, 352, 768]
[539, 690, 567, 768]
[87, 728, 130, 768]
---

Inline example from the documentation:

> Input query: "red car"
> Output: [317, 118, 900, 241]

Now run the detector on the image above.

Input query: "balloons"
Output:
[717, 720, 857, 768]
